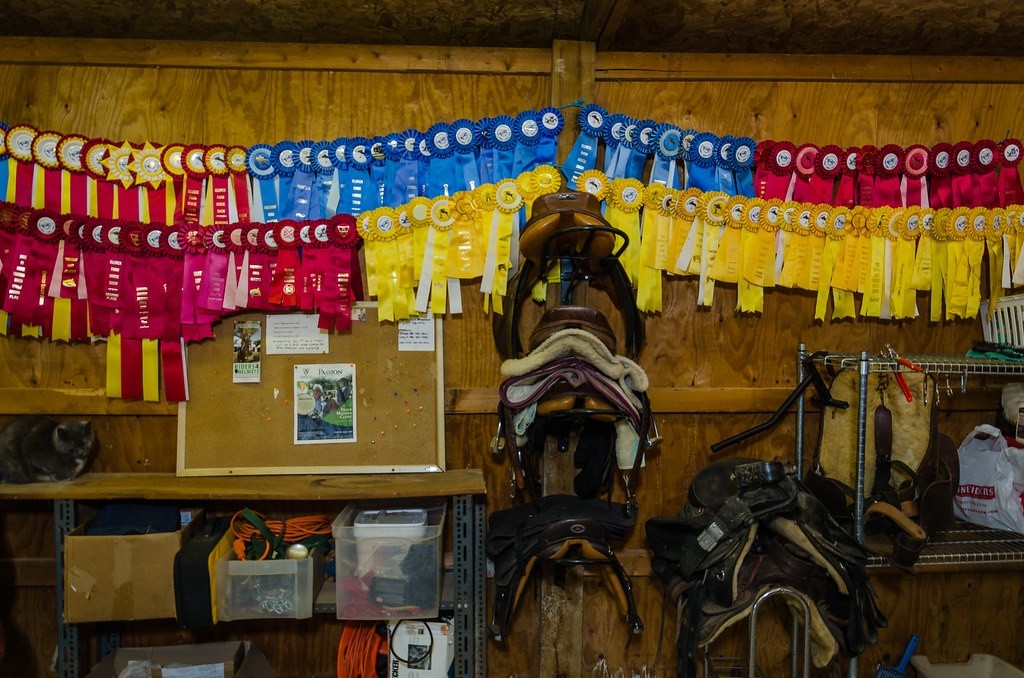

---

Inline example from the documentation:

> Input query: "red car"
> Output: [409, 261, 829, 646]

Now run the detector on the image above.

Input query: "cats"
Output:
[0, 414, 97, 484]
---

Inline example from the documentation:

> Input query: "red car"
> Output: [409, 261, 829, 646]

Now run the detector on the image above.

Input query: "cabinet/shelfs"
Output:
[792, 341, 1024, 678]
[0, 468, 488, 678]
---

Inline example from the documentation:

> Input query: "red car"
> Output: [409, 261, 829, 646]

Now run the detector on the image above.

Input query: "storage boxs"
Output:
[85, 640, 276, 678]
[979, 292, 1024, 351]
[331, 498, 446, 620]
[216, 541, 325, 620]
[386, 612, 454, 678]
[61, 505, 206, 622]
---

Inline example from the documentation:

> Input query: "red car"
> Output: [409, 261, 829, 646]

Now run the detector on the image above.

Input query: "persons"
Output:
[338, 377, 349, 401]
[310, 383, 325, 416]
